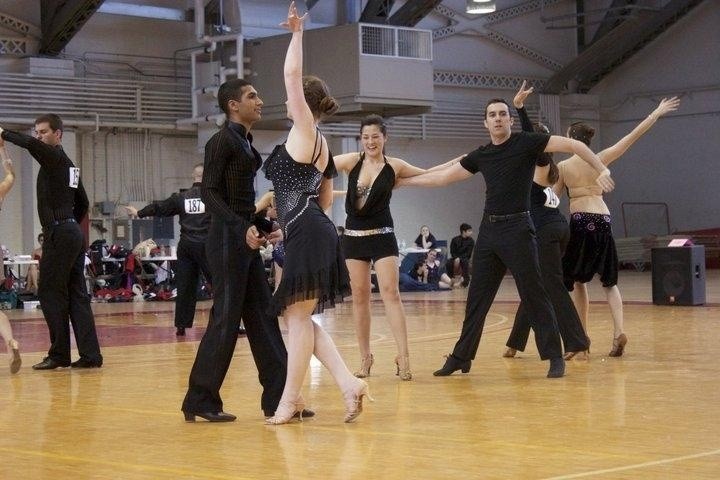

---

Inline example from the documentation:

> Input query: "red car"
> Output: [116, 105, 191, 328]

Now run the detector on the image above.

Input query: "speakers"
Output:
[651, 246, 706, 306]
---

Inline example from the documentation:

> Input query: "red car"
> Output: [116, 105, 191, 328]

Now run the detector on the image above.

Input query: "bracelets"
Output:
[2, 159, 13, 165]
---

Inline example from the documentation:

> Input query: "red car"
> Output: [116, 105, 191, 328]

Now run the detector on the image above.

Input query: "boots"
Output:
[8, 339, 22, 374]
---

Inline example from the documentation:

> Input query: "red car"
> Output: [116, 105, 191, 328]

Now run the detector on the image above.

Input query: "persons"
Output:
[25, 232, 44, 291]
[258, 0, 377, 428]
[0, 135, 16, 373]
[0, 113, 105, 370]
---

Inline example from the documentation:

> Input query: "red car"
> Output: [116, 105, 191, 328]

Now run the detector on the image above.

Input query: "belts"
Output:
[41, 218, 78, 232]
[480, 212, 529, 223]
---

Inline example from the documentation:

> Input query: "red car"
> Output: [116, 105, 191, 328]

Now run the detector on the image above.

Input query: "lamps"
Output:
[464, 1, 497, 14]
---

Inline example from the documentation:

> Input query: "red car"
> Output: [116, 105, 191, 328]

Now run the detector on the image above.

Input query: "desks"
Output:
[368, 245, 444, 291]
[102, 255, 179, 293]
[2, 258, 41, 307]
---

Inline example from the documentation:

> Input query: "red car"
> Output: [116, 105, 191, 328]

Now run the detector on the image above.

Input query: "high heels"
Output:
[343, 380, 373, 424]
[546, 358, 565, 379]
[394, 353, 413, 380]
[354, 353, 376, 378]
[261, 394, 315, 420]
[502, 348, 516, 358]
[608, 333, 628, 358]
[564, 338, 591, 361]
[181, 404, 237, 422]
[262, 395, 315, 424]
[432, 354, 472, 375]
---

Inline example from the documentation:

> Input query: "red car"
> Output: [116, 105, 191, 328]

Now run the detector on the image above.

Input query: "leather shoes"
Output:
[176, 328, 187, 335]
[72, 354, 105, 368]
[33, 356, 70, 371]
